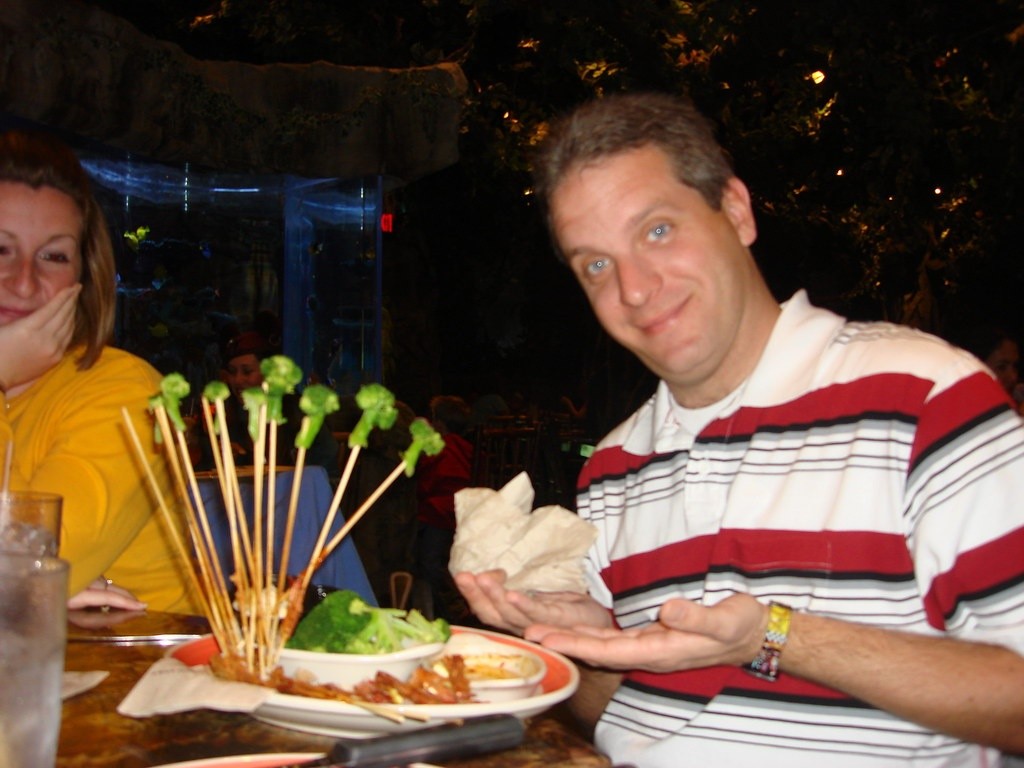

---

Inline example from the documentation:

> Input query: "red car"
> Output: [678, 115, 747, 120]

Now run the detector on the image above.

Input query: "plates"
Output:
[147, 752, 444, 768]
[165, 626, 581, 738]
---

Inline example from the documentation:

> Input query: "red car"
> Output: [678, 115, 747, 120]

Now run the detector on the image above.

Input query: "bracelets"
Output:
[743, 602, 792, 682]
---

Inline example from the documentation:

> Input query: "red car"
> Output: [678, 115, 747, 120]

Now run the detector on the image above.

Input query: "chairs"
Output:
[322, 413, 599, 522]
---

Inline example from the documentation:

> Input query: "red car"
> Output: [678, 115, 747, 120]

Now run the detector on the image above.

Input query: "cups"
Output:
[0, 491, 63, 559]
[1, 552, 69, 767]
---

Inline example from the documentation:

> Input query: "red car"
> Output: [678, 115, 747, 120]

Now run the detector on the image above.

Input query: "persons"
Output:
[0, 141, 206, 620]
[214, 330, 298, 468]
[446, 91, 1024, 765]
[417, 393, 475, 620]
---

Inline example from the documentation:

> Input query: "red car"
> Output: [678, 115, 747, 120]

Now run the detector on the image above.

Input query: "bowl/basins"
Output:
[266, 619, 444, 695]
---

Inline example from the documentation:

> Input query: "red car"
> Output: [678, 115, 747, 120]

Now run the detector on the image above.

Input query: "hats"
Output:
[225, 331, 263, 358]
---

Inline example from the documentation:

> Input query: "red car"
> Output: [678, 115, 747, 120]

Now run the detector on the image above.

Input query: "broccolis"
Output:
[289, 589, 451, 655]
[147, 354, 445, 478]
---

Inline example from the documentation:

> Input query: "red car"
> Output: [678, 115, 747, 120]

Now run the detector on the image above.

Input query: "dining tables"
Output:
[0, 603, 611, 767]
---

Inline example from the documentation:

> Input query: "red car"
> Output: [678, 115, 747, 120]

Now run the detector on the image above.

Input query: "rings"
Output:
[101, 576, 114, 593]
